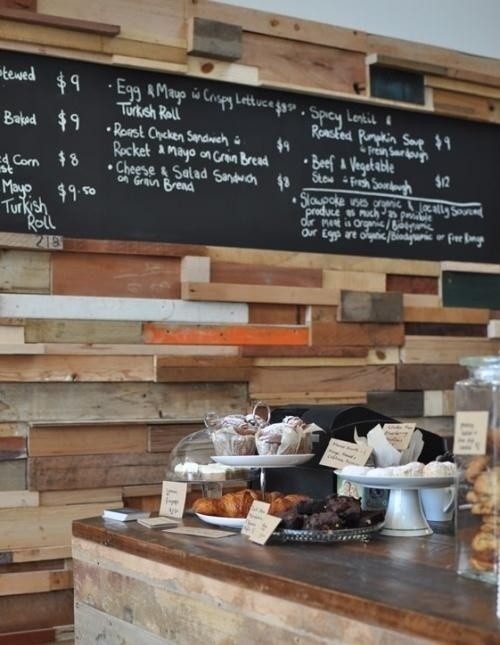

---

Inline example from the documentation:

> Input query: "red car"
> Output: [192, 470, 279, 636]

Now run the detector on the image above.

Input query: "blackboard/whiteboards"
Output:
[0, 39, 499, 277]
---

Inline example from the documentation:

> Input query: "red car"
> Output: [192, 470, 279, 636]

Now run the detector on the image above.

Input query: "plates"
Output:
[195, 512, 246, 530]
[272, 519, 387, 543]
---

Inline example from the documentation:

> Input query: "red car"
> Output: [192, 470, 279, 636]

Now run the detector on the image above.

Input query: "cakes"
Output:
[204, 397, 318, 456]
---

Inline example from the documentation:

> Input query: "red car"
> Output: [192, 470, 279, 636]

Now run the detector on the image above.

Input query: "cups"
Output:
[421, 486, 456, 521]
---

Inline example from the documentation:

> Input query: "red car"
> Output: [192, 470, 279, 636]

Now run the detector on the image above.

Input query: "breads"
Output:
[449, 423, 499, 572]
[192, 488, 307, 517]
[262, 494, 385, 532]
[337, 461, 461, 479]
